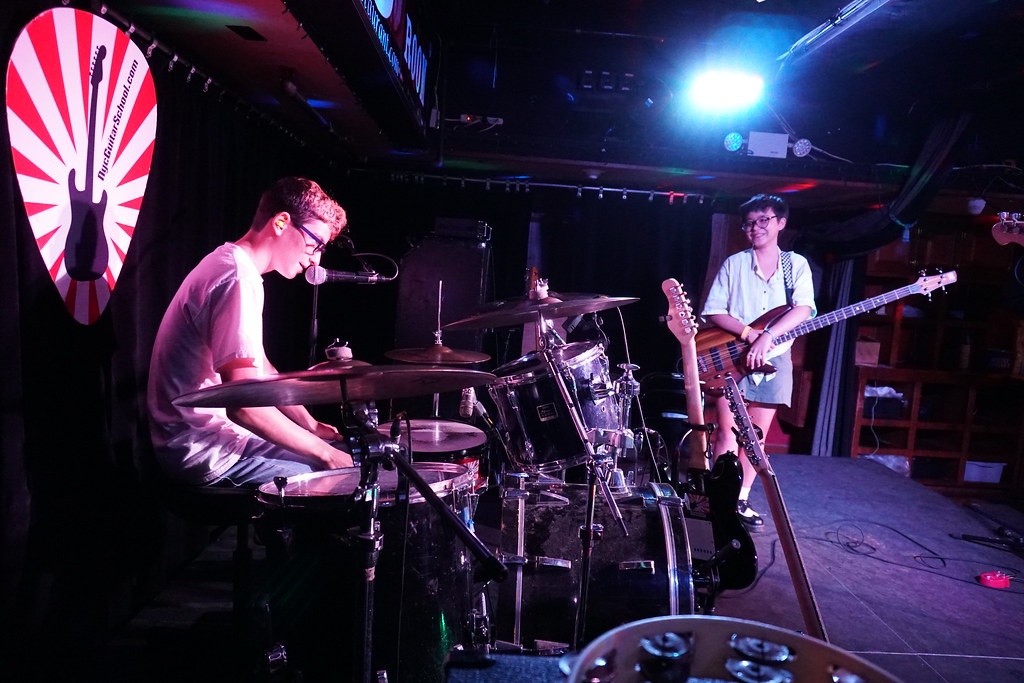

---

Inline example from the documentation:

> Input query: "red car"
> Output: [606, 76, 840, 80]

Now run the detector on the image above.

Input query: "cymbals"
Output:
[169, 363, 497, 407]
[384, 346, 490, 363]
[567, 612, 901, 682]
[441, 296, 641, 333]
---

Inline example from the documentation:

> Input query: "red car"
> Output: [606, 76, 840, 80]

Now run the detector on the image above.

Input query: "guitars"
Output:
[694, 269, 950, 404]
[724, 376, 827, 639]
[659, 278, 758, 593]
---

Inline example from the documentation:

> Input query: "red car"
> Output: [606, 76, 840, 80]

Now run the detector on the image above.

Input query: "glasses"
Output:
[273, 214, 326, 255]
[741, 215, 786, 233]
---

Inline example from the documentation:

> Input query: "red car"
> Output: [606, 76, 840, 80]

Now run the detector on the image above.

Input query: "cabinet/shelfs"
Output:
[840, 363, 1024, 499]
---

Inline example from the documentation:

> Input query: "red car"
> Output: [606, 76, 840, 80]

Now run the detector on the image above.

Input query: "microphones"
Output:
[561, 296, 601, 334]
[305, 266, 391, 286]
[460, 386, 475, 417]
[382, 415, 402, 471]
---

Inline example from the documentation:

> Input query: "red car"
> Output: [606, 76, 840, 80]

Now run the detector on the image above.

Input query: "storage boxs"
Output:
[964, 460, 1007, 484]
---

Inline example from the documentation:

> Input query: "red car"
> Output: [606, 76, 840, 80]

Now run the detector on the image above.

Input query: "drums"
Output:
[487, 339, 619, 473]
[496, 480, 693, 653]
[250, 460, 473, 683]
[379, 416, 486, 459]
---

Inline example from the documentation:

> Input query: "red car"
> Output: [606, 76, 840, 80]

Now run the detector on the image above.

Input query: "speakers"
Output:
[684, 517, 715, 565]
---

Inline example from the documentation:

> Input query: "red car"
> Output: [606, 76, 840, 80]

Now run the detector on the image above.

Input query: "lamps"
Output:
[723, 131, 812, 159]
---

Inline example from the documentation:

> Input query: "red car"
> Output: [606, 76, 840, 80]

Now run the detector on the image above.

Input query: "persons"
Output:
[149, 176, 352, 502]
[699, 193, 817, 530]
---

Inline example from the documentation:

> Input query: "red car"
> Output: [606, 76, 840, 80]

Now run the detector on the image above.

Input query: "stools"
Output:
[191, 483, 263, 595]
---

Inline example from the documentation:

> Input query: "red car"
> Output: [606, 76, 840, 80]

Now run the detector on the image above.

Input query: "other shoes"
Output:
[737, 500, 765, 533]
